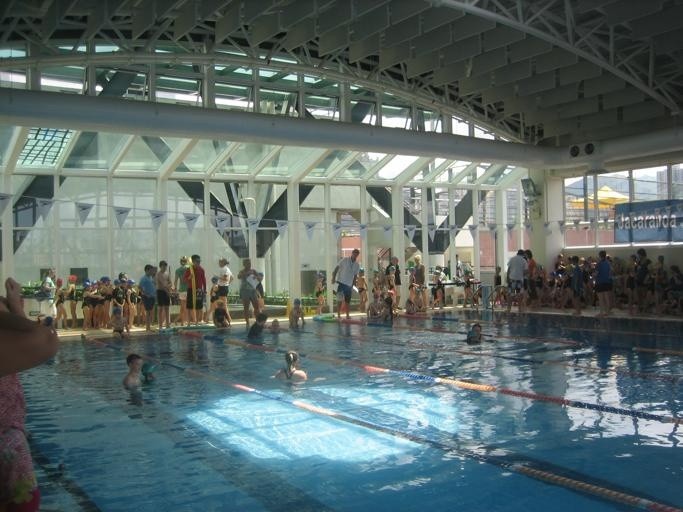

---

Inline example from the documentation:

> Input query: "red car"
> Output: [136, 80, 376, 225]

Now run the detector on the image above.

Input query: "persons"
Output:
[274, 350, 307, 381]
[495, 249, 680, 317]
[0, 312, 58, 512]
[329, 244, 483, 321]
[40, 254, 326, 343]
[140, 363, 158, 383]
[0, 277, 29, 319]
[121, 352, 143, 389]
[464, 324, 486, 345]
